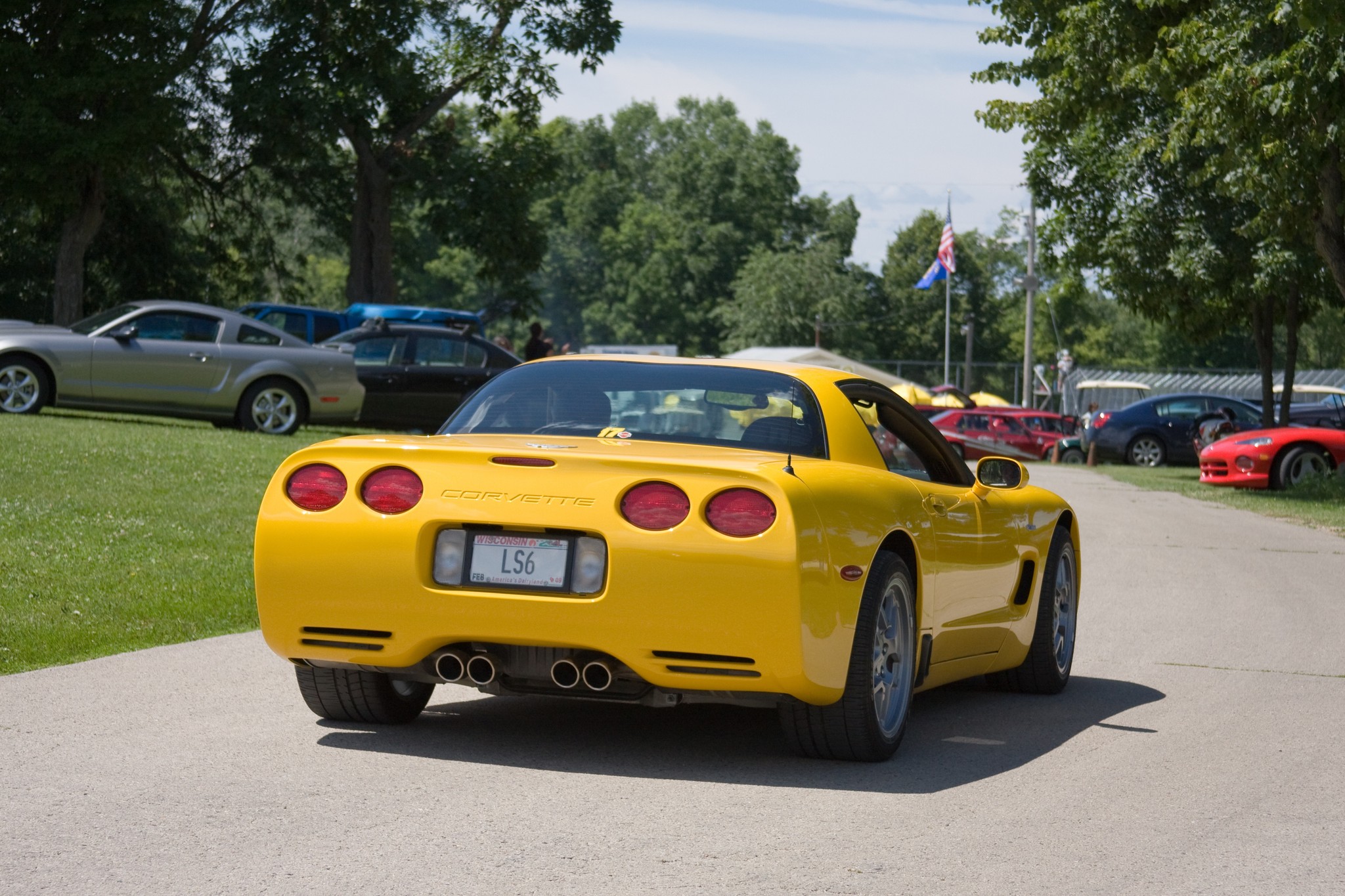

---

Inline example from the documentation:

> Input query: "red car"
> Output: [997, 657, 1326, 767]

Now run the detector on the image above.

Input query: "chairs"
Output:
[740, 417, 815, 457]
[531, 386, 613, 436]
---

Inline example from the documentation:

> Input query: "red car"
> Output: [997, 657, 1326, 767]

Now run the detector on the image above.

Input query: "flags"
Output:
[914, 194, 957, 291]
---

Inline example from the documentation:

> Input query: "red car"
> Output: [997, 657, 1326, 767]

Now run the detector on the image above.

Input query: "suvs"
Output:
[225, 302, 527, 430]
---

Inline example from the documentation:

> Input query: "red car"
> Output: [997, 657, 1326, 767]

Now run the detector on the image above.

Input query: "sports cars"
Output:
[1197, 424, 1345, 494]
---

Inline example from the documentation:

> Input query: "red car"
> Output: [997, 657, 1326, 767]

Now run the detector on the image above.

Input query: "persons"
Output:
[552, 385, 612, 425]
[1188, 406, 1236, 460]
[492, 322, 571, 363]
[1076, 402, 1101, 465]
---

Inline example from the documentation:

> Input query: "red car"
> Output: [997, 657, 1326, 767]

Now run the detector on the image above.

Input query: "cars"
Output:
[872, 393, 1345, 468]
[0, 300, 364, 436]
[252, 354, 1085, 766]
